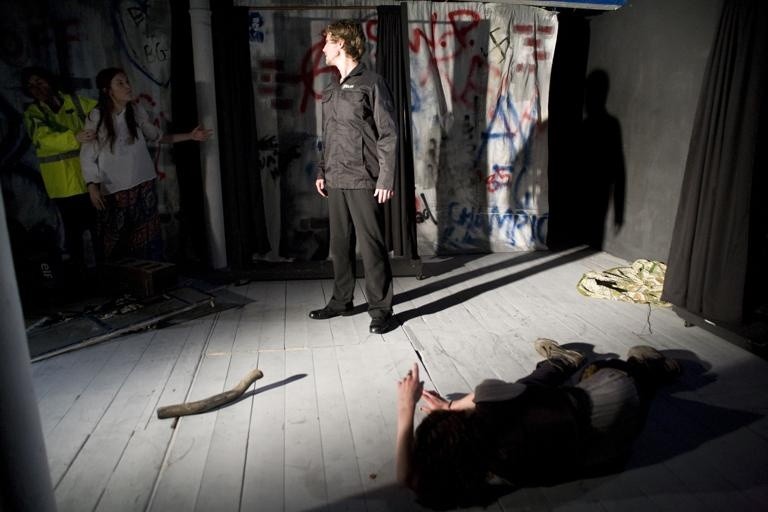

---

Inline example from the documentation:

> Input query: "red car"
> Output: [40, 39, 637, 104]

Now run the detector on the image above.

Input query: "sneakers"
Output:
[535, 338, 585, 368]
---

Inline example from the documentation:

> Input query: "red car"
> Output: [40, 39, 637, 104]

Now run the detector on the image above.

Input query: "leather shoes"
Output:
[369, 320, 391, 334]
[309, 302, 353, 319]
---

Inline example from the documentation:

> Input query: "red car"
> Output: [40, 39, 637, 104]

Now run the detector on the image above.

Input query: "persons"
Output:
[395, 337, 680, 498]
[309, 19, 398, 334]
